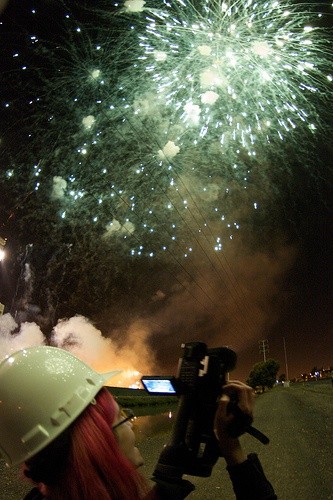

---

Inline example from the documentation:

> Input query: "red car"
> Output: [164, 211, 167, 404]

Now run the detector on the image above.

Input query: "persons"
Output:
[20, 380, 278, 500]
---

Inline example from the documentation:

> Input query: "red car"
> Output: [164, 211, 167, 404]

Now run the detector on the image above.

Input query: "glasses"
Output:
[105, 408, 136, 429]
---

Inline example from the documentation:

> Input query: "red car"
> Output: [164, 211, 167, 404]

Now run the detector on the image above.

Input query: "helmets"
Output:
[0, 346, 124, 470]
